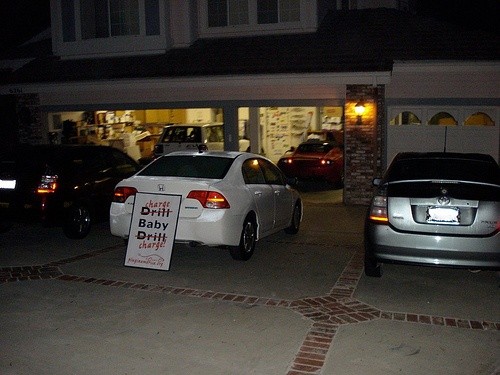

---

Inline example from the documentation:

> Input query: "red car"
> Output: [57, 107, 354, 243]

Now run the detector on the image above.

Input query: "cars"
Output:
[0, 145, 143, 241]
[108, 150, 305, 260]
[277, 129, 345, 192]
[362, 151, 500, 278]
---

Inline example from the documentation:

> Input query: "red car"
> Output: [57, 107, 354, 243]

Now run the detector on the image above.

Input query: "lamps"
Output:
[355, 97, 365, 115]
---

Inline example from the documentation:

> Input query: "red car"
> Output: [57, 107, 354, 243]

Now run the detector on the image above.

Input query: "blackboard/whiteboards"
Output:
[123, 192, 183, 272]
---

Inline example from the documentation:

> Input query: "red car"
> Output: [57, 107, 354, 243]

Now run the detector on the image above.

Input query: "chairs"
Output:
[177, 165, 199, 176]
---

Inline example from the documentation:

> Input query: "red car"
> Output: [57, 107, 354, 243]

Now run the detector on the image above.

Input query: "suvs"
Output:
[150, 120, 264, 161]
